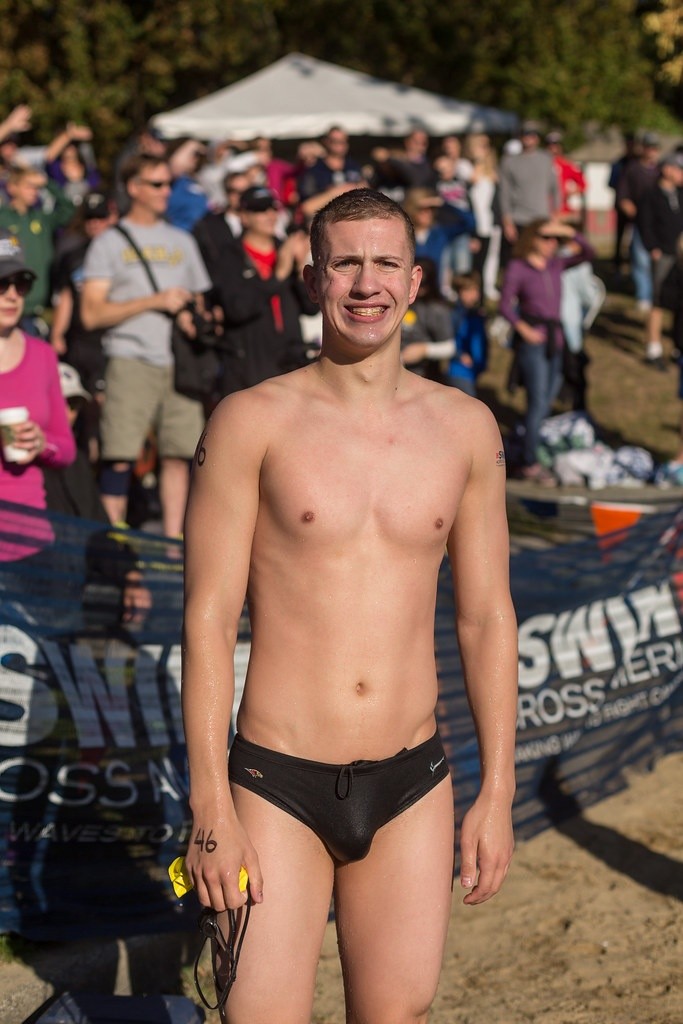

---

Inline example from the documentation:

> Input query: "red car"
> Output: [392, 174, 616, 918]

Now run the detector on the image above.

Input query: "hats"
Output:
[0, 228, 34, 279]
[241, 185, 280, 213]
[81, 190, 112, 220]
[57, 361, 93, 403]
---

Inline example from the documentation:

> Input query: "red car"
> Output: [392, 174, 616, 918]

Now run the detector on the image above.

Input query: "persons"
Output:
[0, 227, 79, 591]
[41, 360, 153, 648]
[78, 149, 220, 563]
[547, 222, 607, 414]
[180, 187, 518, 1024]
[190, 169, 253, 313]
[48, 186, 120, 408]
[0, 167, 77, 339]
[216, 184, 323, 396]
[500, 214, 599, 488]
[0, 103, 500, 300]
[490, 116, 683, 399]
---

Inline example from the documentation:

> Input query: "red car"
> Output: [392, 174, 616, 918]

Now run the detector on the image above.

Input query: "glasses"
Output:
[67, 397, 82, 410]
[138, 180, 175, 188]
[0, 271, 34, 297]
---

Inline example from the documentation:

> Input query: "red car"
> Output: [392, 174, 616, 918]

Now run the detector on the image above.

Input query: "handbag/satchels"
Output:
[171, 320, 230, 402]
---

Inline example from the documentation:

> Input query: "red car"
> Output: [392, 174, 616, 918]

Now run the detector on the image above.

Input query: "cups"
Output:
[0, 407, 31, 461]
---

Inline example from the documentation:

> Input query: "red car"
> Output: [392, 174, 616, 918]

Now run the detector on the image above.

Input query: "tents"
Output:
[150, 50, 519, 142]
